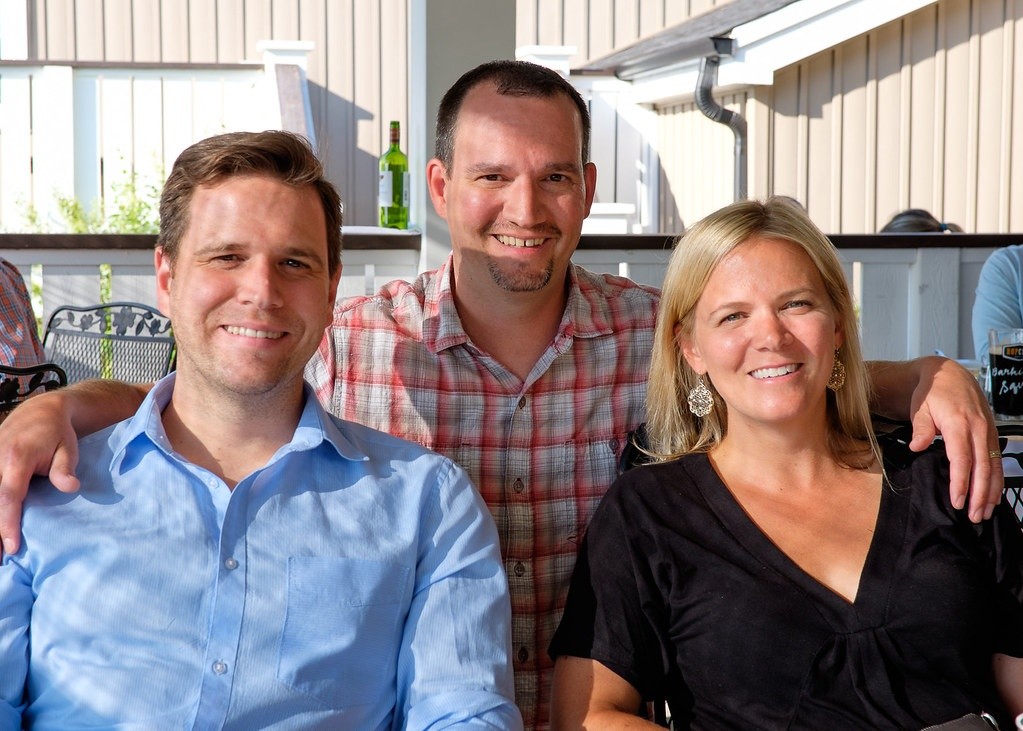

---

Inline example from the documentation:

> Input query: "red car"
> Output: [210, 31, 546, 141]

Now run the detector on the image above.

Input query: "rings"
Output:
[989, 450, 1002, 458]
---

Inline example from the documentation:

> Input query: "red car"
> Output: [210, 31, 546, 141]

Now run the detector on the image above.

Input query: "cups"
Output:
[987, 328, 1023, 423]
[948, 356, 983, 390]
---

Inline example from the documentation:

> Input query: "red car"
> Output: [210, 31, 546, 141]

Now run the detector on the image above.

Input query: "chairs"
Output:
[0, 364, 67, 416]
[40, 301, 174, 386]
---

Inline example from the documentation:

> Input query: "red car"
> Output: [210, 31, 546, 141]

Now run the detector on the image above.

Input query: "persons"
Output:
[878, 208, 965, 234]
[0, 261, 50, 425]
[0, 60, 1005, 731]
[971, 245, 1023, 380]
[540, 194, 1023, 731]
[0, 132, 522, 731]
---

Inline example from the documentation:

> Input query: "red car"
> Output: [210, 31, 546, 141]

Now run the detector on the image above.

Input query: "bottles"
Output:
[378, 120, 408, 230]
